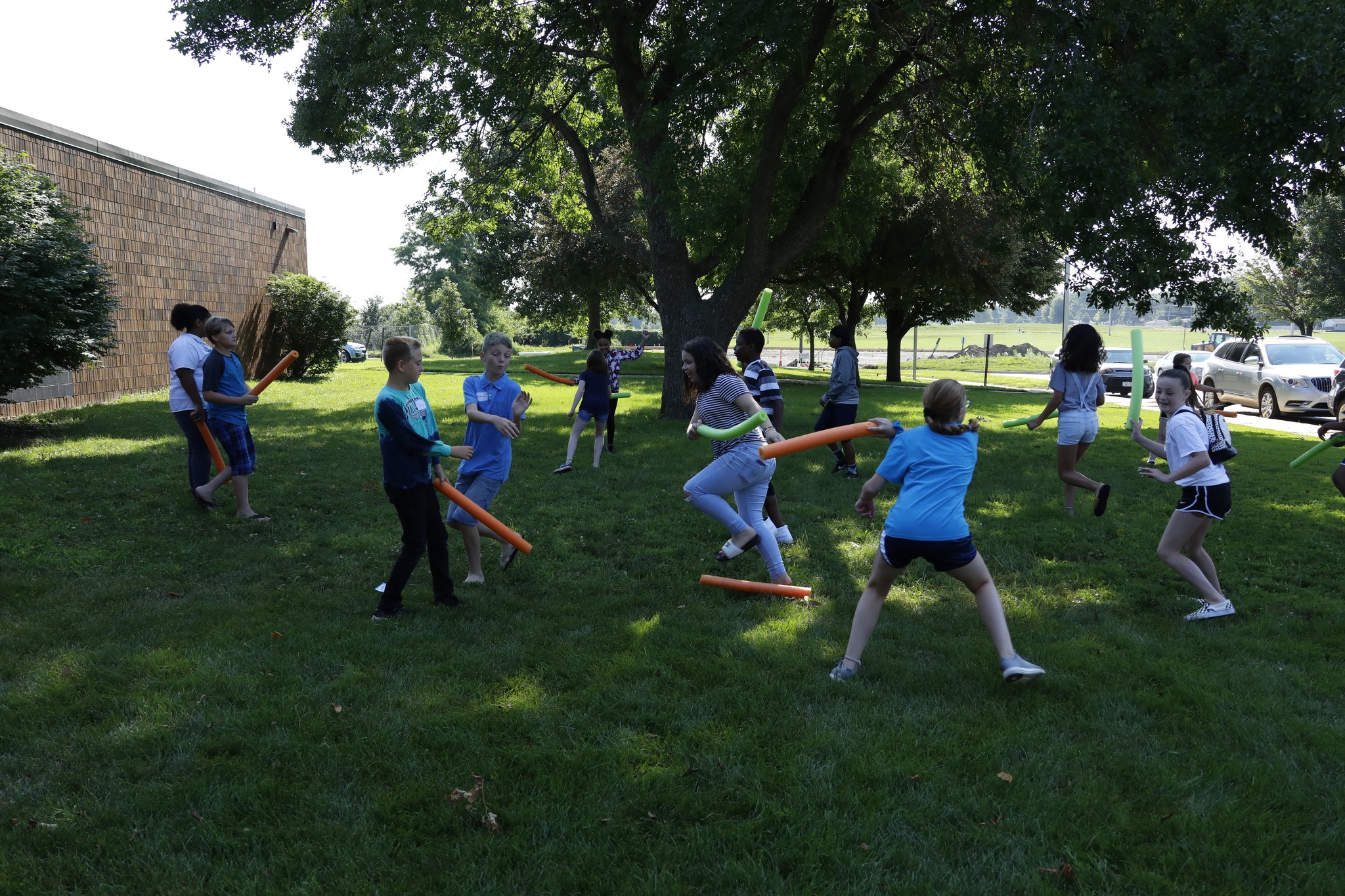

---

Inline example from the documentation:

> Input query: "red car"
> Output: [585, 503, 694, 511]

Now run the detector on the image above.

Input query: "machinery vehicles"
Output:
[1191, 331, 1238, 352]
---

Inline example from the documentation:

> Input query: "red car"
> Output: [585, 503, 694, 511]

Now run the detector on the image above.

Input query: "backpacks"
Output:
[1171, 405, 1236, 464]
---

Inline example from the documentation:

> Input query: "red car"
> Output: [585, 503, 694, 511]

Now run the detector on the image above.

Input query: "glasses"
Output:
[955, 399, 972, 416]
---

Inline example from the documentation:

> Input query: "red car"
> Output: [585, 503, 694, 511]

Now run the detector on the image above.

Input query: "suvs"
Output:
[1049, 346, 1155, 398]
[1200, 335, 1345, 419]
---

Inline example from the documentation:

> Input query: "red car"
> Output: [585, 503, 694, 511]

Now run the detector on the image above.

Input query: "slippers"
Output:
[501, 533, 525, 573]
[240, 513, 272, 523]
[190, 488, 216, 514]
[714, 535, 761, 561]
[1092, 484, 1110, 518]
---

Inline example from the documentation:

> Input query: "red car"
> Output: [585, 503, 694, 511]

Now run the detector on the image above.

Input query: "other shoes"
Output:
[195, 496, 220, 506]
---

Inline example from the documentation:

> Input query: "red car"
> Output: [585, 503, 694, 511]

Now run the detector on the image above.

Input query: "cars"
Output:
[333, 338, 368, 363]
[1327, 356, 1345, 423]
[1154, 350, 1213, 384]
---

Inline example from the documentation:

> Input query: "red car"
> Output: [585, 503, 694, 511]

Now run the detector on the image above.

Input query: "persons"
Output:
[829, 378, 1046, 684]
[1317, 420, 1345, 498]
[167, 303, 217, 511]
[1146, 353, 1224, 465]
[1124, 364, 1235, 621]
[566, 329, 649, 455]
[371, 335, 474, 621]
[814, 326, 859, 480]
[682, 337, 792, 586]
[191, 316, 271, 523]
[1026, 324, 1111, 517]
[553, 349, 612, 475]
[446, 331, 533, 584]
[733, 327, 794, 544]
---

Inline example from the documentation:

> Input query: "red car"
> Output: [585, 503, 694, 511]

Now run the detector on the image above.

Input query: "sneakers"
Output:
[606, 444, 616, 457]
[431, 597, 467, 608]
[831, 460, 849, 475]
[1001, 647, 1046, 686]
[844, 471, 859, 480]
[775, 525, 794, 545]
[1183, 599, 1235, 622]
[1188, 596, 1228, 605]
[1145, 460, 1155, 465]
[829, 656, 858, 685]
[554, 462, 572, 475]
[764, 515, 778, 536]
[373, 604, 416, 620]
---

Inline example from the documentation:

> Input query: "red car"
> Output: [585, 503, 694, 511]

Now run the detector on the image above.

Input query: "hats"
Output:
[830, 324, 851, 340]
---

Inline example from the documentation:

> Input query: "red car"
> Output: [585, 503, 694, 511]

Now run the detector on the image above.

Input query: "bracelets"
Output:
[889, 421, 904, 443]
[764, 428, 776, 436]
[763, 426, 776, 433]
[1036, 418, 1041, 425]
